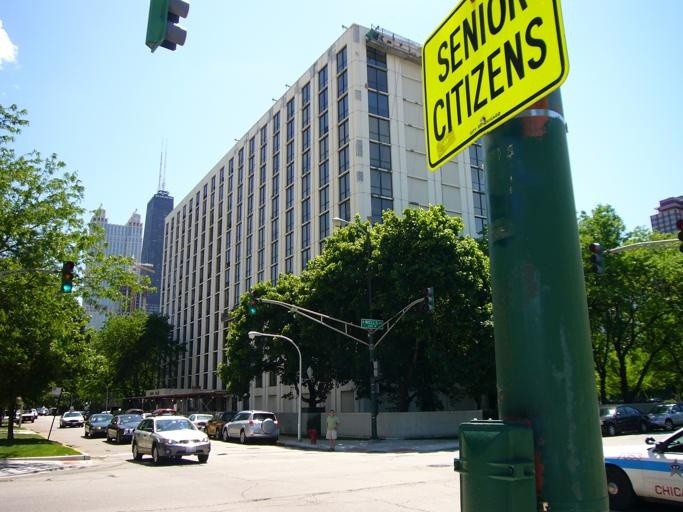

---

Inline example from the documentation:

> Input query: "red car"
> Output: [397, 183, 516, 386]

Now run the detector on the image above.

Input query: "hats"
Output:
[2, 405, 279, 466]
[600, 398, 683, 512]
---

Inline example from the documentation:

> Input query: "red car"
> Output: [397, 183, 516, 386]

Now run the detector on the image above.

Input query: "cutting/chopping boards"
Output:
[308, 428, 317, 444]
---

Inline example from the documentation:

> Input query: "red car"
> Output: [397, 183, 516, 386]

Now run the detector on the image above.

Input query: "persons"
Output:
[325, 410, 340, 451]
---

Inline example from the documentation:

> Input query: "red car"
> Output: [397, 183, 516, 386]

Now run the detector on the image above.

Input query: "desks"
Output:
[248, 331, 302, 440]
[332, 216, 379, 440]
[85, 379, 108, 411]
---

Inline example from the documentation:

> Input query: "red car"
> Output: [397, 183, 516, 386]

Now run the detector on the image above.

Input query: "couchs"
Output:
[588, 243, 603, 274]
[144, 0, 189, 51]
[676, 218, 683, 253]
[423, 287, 435, 312]
[62, 260, 74, 293]
[250, 289, 257, 315]
